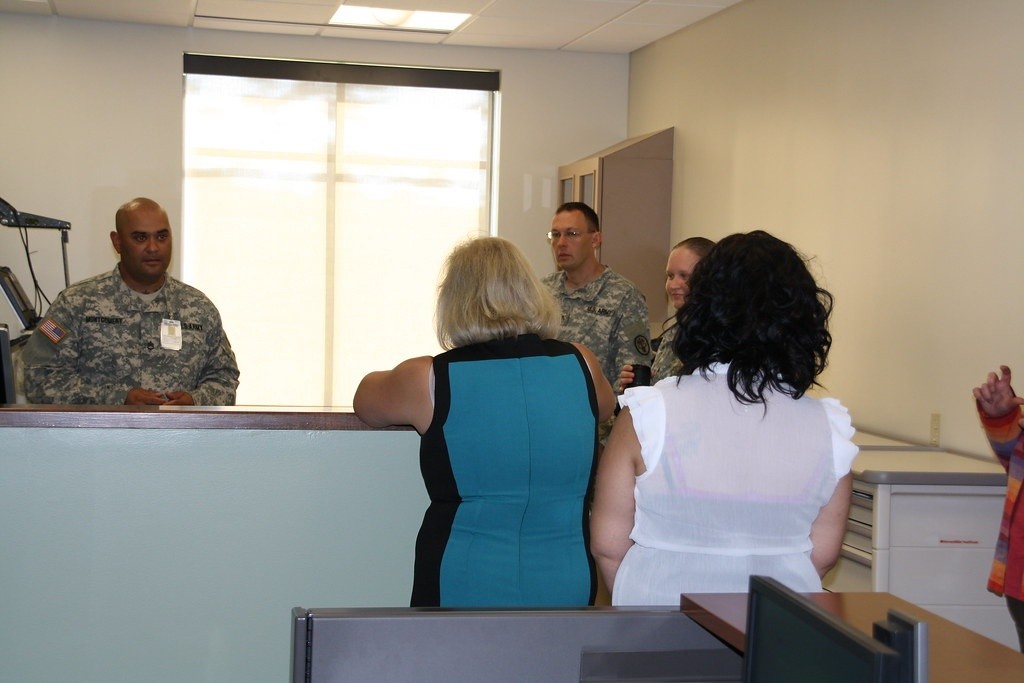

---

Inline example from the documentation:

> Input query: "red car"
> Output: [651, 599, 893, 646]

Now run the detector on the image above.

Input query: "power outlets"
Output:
[929, 415, 942, 449]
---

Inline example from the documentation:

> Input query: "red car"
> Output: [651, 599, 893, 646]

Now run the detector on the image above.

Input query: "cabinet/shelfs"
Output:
[827, 427, 1024, 655]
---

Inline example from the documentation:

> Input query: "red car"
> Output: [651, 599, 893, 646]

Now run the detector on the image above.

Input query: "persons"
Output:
[537, 202, 653, 461]
[588, 230, 860, 606]
[353, 237, 618, 608]
[22, 198, 240, 406]
[971, 365, 1024, 655]
[618, 237, 716, 394]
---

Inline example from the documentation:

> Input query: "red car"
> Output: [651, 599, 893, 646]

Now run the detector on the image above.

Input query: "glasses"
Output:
[546, 230, 596, 242]
